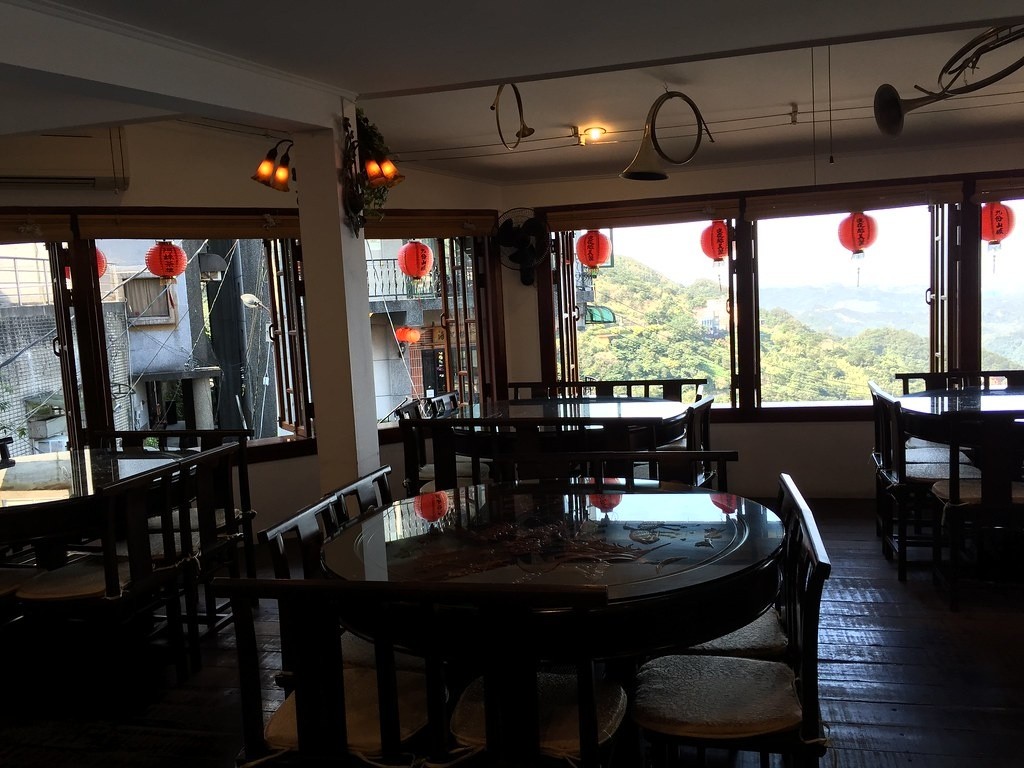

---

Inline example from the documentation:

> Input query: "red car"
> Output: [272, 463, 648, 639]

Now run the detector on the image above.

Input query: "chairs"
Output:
[259, 492, 344, 700]
[930, 411, 1024, 609]
[979, 369, 1024, 389]
[0, 427, 258, 722]
[630, 508, 832, 768]
[404, 582, 608, 768]
[637, 472, 810, 663]
[613, 450, 739, 492]
[493, 452, 614, 483]
[324, 464, 397, 531]
[879, 396, 980, 582]
[394, 378, 715, 497]
[212, 576, 404, 768]
[895, 371, 980, 459]
[866, 380, 976, 562]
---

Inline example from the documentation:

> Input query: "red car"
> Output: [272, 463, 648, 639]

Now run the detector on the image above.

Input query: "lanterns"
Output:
[65, 246, 108, 279]
[145, 240, 188, 284]
[588, 475, 623, 514]
[981, 200, 1015, 246]
[838, 212, 877, 255]
[700, 219, 729, 262]
[395, 327, 422, 344]
[414, 491, 449, 523]
[708, 493, 745, 513]
[576, 230, 611, 272]
[398, 240, 433, 285]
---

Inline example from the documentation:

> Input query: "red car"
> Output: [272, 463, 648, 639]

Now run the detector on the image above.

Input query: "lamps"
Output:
[349, 140, 406, 186]
[251, 139, 293, 193]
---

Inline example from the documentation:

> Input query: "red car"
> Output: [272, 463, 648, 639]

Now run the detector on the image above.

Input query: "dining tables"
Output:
[896, 386, 1024, 449]
[0, 446, 202, 570]
[320, 476, 786, 658]
[436, 396, 689, 452]
